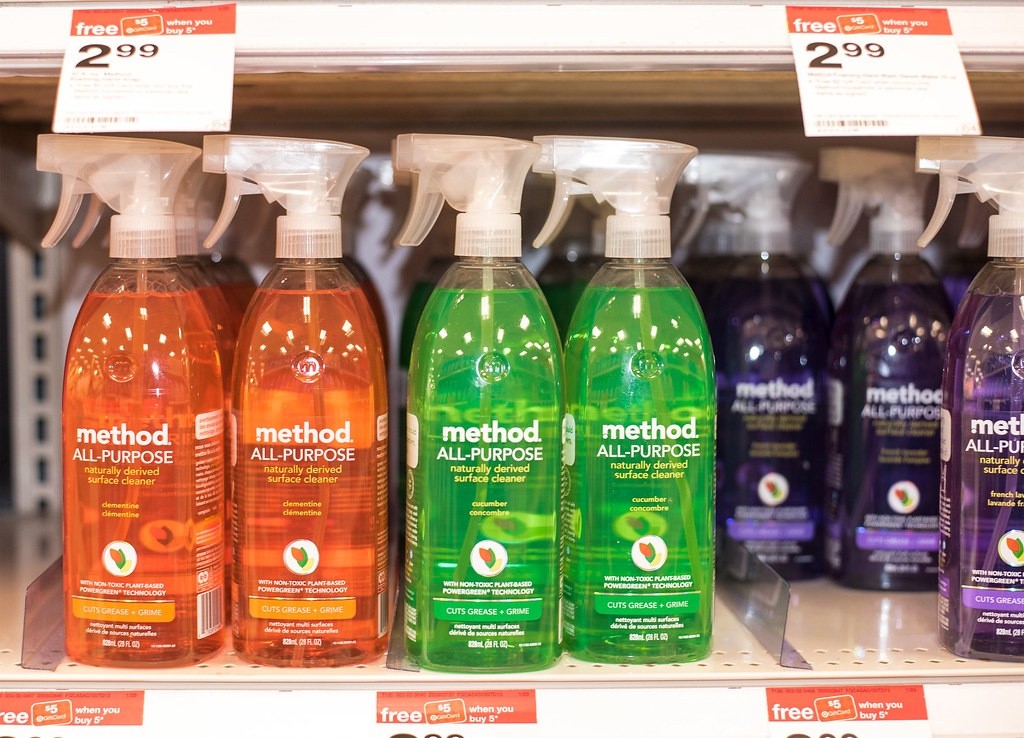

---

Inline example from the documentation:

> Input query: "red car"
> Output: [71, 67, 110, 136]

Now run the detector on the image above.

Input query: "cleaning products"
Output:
[27, 126, 1024, 674]
